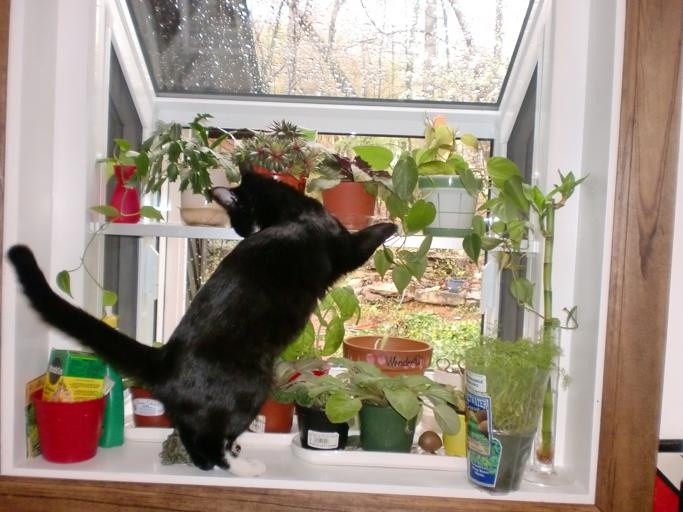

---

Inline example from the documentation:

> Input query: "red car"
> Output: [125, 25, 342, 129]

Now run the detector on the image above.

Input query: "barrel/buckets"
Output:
[358, 396, 419, 453]
[464, 351, 542, 494]
[294, 401, 349, 450]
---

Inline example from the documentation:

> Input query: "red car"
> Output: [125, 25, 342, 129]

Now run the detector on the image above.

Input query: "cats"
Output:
[6, 157, 398, 479]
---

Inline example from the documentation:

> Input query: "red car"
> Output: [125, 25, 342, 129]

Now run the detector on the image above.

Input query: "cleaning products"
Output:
[99, 307, 123, 449]
